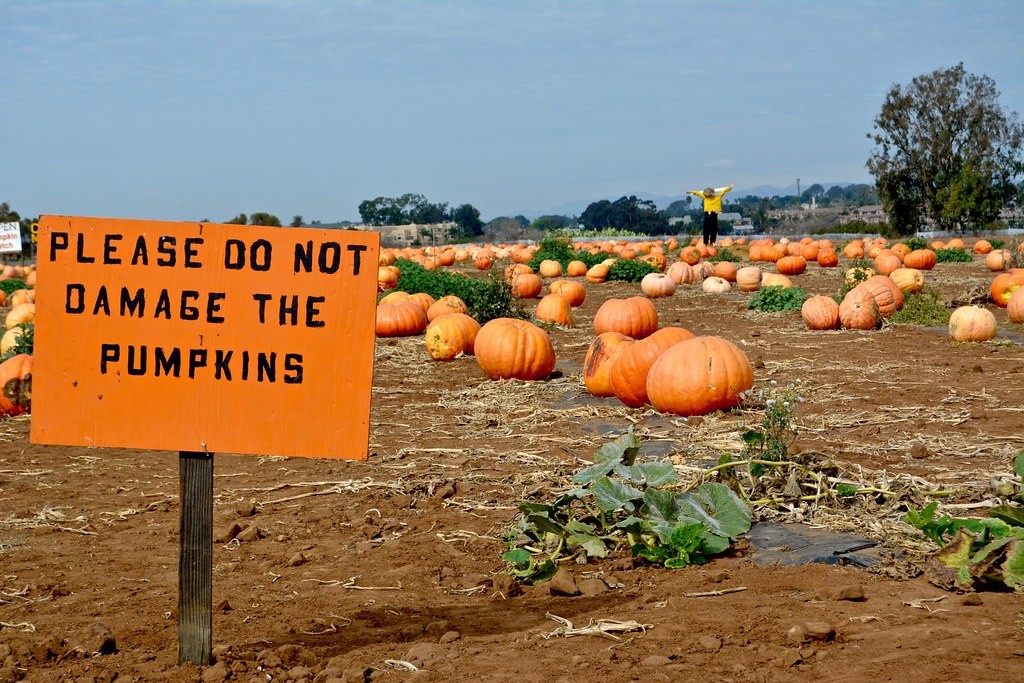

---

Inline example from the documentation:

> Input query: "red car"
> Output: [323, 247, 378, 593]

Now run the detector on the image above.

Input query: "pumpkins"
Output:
[0, 263, 37, 418]
[376, 237, 1024, 416]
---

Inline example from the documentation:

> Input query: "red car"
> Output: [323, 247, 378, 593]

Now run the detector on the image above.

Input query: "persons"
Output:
[686, 182, 735, 246]
